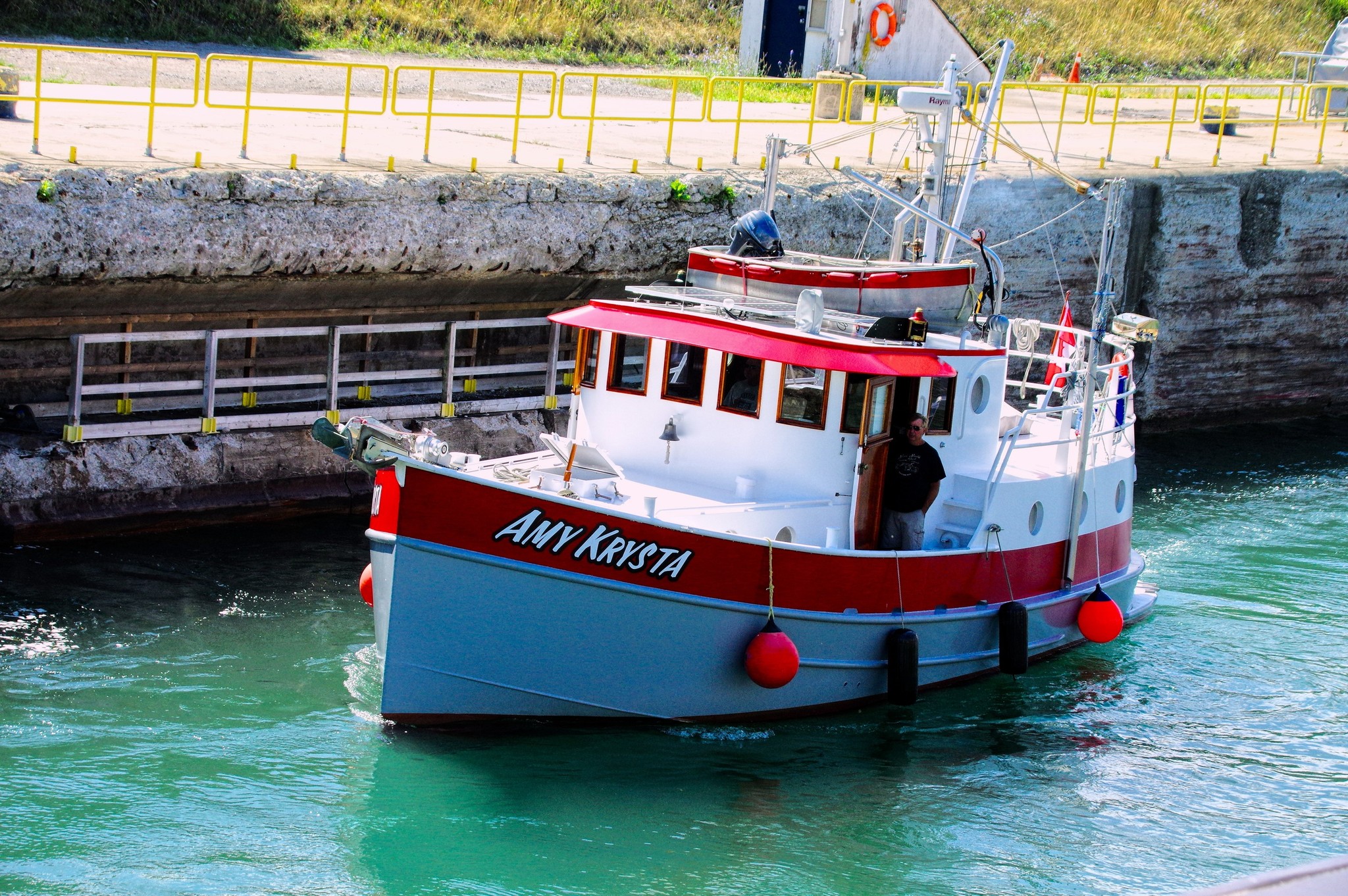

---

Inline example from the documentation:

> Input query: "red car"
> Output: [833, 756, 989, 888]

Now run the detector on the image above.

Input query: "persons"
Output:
[879, 413, 946, 552]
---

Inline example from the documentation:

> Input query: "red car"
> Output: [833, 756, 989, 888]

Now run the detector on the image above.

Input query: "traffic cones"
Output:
[1029, 50, 1045, 82]
[1066, 51, 1082, 83]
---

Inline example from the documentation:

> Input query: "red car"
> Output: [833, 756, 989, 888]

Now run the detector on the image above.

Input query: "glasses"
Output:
[906, 424, 927, 431]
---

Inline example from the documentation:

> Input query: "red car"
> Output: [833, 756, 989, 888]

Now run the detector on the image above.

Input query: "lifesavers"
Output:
[869, 3, 897, 48]
[1107, 353, 1129, 384]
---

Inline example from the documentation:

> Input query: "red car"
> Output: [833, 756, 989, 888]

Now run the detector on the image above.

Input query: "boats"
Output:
[309, 31, 1162, 741]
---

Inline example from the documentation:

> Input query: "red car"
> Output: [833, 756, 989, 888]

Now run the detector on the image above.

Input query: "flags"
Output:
[1101, 353, 1129, 434]
[1044, 300, 1077, 388]
